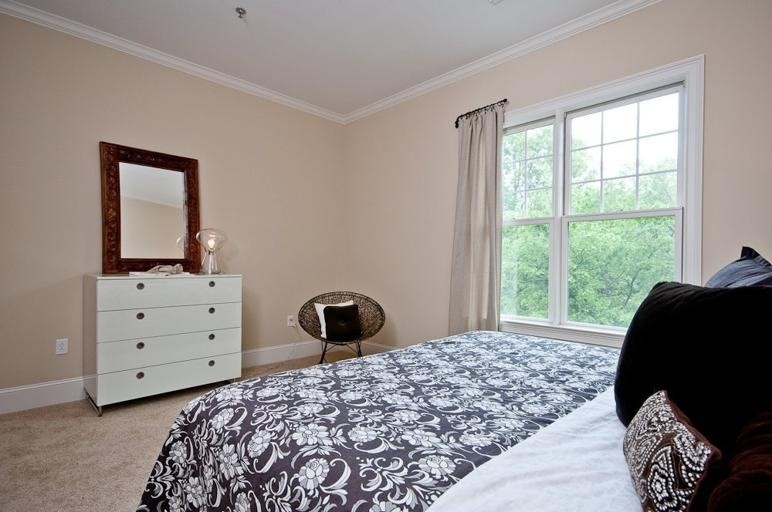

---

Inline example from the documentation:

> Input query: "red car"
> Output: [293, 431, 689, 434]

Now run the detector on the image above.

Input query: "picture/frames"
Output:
[98, 141, 200, 275]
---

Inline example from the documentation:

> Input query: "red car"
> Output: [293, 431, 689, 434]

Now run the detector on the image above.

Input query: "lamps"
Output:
[196, 229, 228, 274]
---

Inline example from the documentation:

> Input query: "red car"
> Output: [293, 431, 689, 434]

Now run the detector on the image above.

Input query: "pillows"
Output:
[690, 420, 770, 511]
[618, 279, 770, 463]
[618, 388, 723, 511]
[702, 245, 769, 289]
[313, 299, 362, 340]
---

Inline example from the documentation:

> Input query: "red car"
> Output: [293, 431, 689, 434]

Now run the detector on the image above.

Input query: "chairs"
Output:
[299, 291, 386, 363]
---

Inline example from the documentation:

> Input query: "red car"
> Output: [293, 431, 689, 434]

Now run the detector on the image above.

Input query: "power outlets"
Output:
[288, 316, 296, 327]
[56, 338, 69, 354]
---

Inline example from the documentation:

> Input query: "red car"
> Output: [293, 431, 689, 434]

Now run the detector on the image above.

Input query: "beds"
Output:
[169, 329, 771, 512]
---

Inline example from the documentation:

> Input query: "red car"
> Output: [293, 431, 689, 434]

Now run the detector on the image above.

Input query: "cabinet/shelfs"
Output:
[82, 272, 242, 415]
